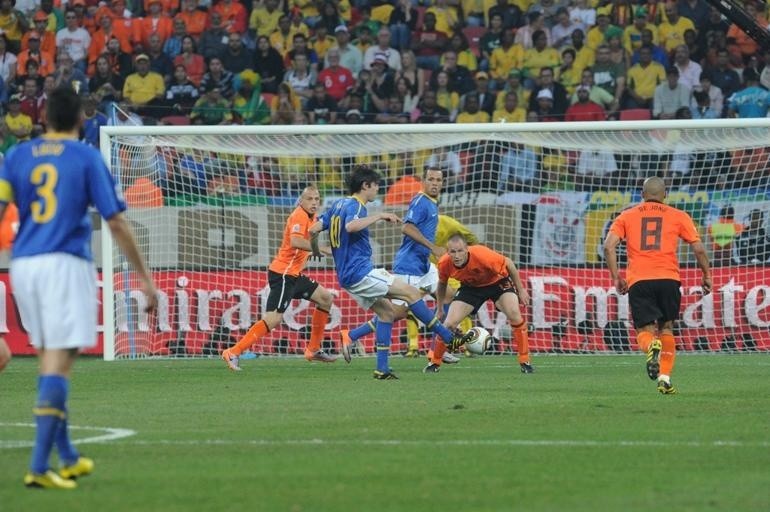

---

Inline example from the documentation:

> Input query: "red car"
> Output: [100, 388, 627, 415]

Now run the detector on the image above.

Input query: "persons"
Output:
[306, 163, 475, 381]
[221, 185, 339, 375]
[707, 207, 768, 266]
[600, 174, 714, 397]
[1, 1, 768, 205]
[400, 213, 479, 361]
[421, 230, 537, 376]
[1, 202, 20, 268]
[340, 162, 462, 369]
[1, 82, 161, 491]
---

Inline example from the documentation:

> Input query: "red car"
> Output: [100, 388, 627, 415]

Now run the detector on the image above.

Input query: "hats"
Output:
[135, 54, 149, 60]
[26, 31, 41, 40]
[370, 52, 390, 67]
[475, 69, 554, 98]
[334, 25, 348, 34]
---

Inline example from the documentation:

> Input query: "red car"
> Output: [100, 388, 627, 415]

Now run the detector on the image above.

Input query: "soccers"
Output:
[465, 326, 490, 354]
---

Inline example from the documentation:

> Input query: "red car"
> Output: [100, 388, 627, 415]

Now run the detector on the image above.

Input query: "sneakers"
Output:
[21, 470, 80, 491]
[656, 378, 675, 394]
[405, 331, 474, 374]
[373, 371, 398, 381]
[222, 348, 243, 372]
[303, 347, 338, 364]
[520, 362, 534, 372]
[59, 456, 94, 476]
[645, 338, 662, 381]
[340, 329, 354, 363]
